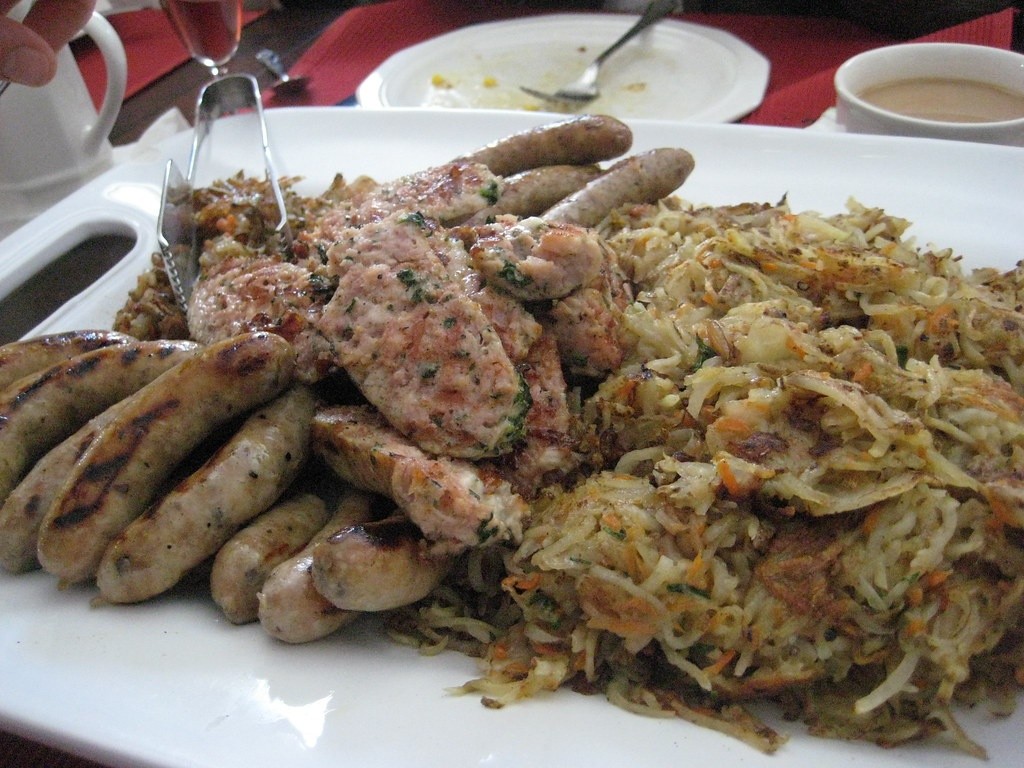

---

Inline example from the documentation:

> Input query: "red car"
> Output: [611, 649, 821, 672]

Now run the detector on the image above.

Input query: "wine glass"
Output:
[160, 0, 244, 117]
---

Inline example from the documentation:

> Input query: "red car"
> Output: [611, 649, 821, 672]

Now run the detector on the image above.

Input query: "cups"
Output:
[0, 0, 128, 220]
[833, 41, 1024, 147]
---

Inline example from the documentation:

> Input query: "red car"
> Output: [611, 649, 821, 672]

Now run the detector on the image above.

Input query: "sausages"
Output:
[0, 325, 453, 643]
[454, 114, 697, 227]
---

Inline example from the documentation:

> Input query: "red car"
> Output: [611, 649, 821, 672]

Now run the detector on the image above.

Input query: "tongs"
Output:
[156, 74, 294, 318]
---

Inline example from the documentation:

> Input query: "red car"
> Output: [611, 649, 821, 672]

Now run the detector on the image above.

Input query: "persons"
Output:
[0, 0, 96, 87]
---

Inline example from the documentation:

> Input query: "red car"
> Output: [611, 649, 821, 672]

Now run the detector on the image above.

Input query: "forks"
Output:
[521, 0, 684, 103]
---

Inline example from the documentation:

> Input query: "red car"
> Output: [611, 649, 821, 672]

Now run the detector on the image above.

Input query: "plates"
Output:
[353, 11, 765, 122]
[0, 108, 1024, 768]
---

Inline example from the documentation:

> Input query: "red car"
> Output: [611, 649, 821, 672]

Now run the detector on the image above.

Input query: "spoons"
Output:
[256, 43, 309, 96]
